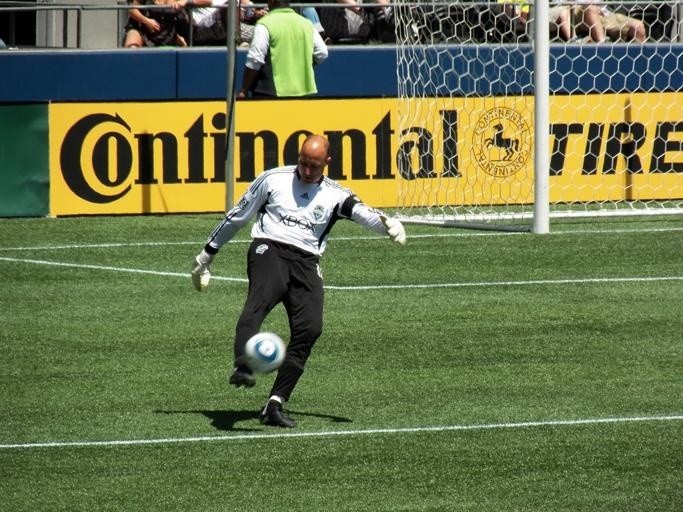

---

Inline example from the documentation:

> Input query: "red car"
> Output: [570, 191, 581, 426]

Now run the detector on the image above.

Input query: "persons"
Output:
[237, 1, 328, 98]
[123, 1, 426, 47]
[190, 135, 406, 427]
[499, 0, 648, 46]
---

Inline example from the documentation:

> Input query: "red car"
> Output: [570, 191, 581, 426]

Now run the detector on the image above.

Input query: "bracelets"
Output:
[240, 88, 248, 96]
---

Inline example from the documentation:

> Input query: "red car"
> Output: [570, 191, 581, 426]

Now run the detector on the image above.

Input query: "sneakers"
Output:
[259, 404, 295, 427]
[229, 367, 256, 389]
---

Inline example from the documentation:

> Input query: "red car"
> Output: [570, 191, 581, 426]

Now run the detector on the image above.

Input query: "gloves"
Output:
[191, 259, 210, 291]
[379, 214, 405, 246]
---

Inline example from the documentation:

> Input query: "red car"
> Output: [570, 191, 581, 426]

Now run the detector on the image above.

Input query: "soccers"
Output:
[245, 331, 287, 375]
